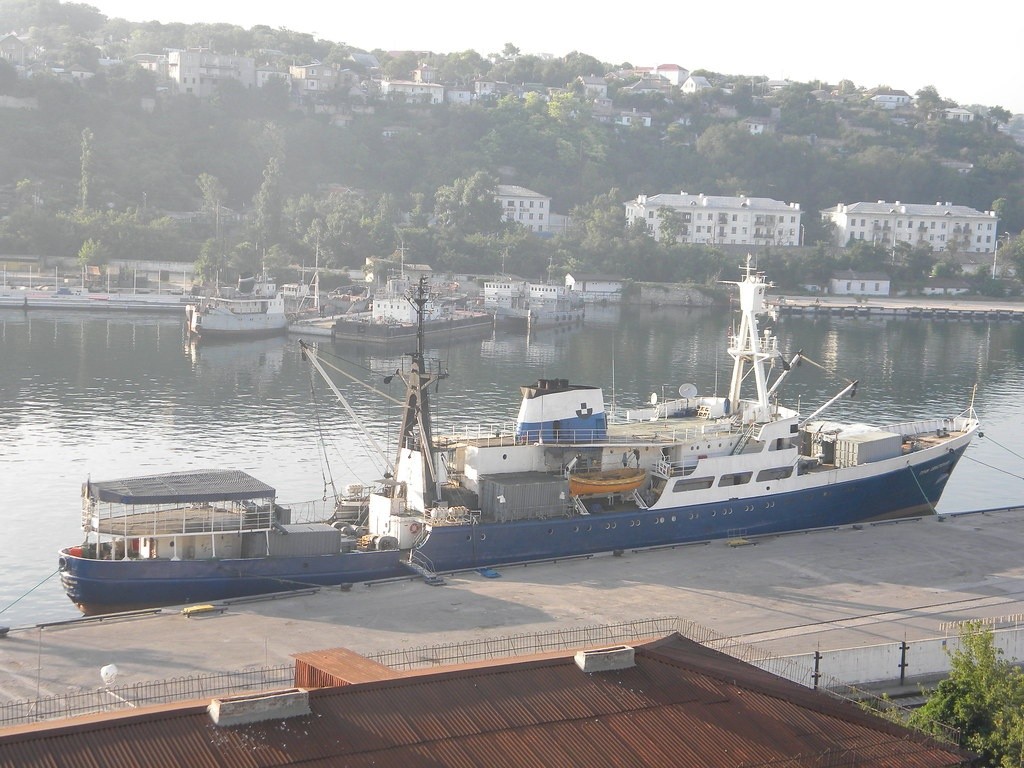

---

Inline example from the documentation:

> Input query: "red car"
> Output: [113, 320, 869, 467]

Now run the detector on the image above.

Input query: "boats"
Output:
[186, 261, 288, 337]
[57, 253, 984, 621]
[568, 467, 646, 496]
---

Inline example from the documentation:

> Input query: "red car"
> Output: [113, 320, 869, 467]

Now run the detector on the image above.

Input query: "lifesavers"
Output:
[410, 523, 419, 534]
